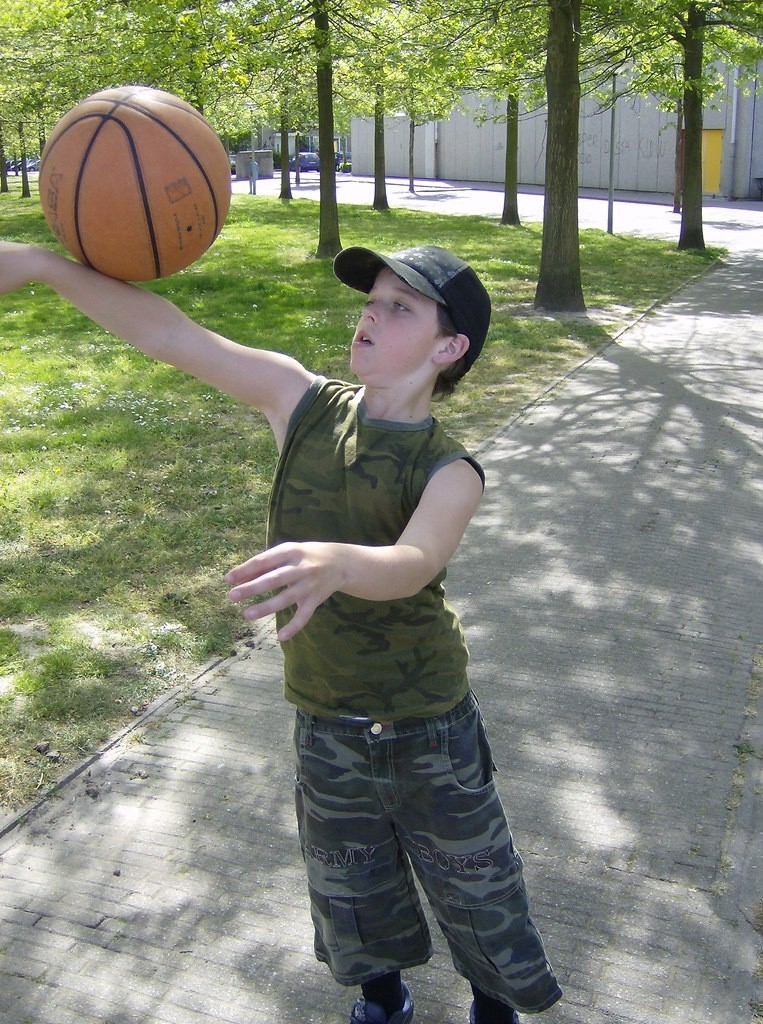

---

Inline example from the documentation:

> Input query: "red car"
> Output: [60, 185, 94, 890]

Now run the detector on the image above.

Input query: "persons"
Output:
[248, 157, 258, 194]
[0, 240, 562, 1024]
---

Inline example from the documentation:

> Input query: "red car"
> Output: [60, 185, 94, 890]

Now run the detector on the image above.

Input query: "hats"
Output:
[333, 245, 491, 373]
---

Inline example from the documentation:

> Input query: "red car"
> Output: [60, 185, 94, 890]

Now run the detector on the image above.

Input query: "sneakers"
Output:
[469, 999, 519, 1024]
[348, 982, 413, 1024]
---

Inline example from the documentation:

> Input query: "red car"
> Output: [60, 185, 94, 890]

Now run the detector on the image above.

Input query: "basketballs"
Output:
[37, 85, 232, 283]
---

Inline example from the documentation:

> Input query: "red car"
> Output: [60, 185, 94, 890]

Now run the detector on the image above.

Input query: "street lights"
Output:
[607, 62, 620, 235]
[245, 101, 257, 194]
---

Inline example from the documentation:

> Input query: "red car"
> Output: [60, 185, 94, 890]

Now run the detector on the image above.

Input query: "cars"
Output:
[229, 155, 236, 174]
[335, 152, 352, 172]
[5, 159, 40, 172]
[289, 152, 320, 172]
